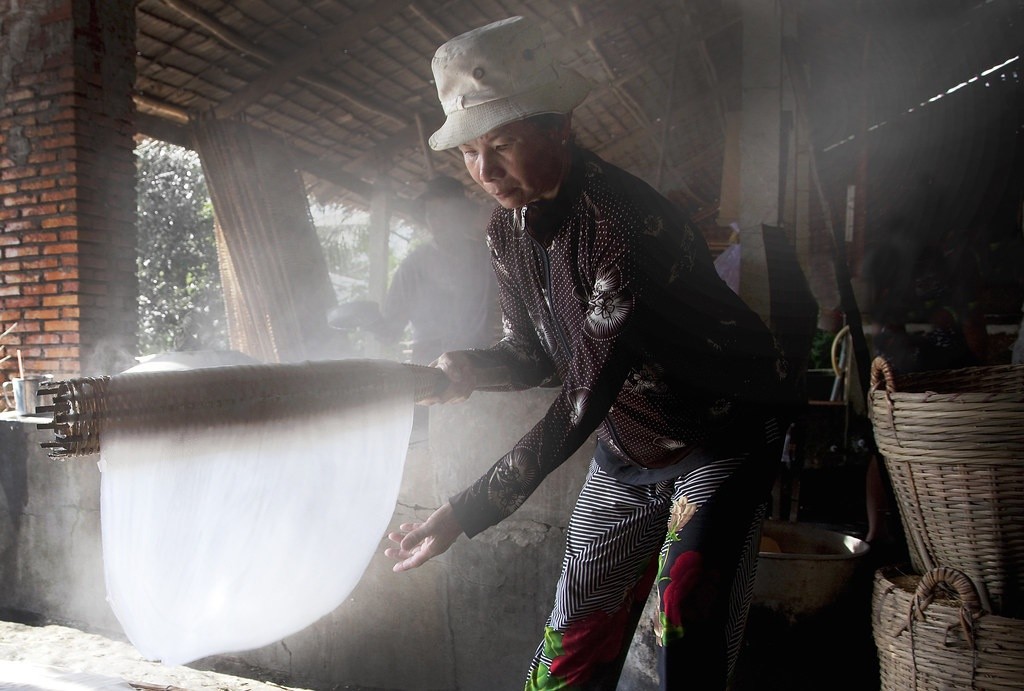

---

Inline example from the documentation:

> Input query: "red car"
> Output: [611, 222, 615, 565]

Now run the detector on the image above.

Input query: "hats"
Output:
[414, 175, 465, 202]
[427, 15, 589, 151]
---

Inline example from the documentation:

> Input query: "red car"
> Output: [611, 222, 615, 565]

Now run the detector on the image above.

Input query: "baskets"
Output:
[868, 357, 1024, 598]
[869, 569, 1024, 691]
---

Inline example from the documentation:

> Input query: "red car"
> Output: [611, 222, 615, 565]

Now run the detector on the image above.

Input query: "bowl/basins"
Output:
[751, 519, 870, 617]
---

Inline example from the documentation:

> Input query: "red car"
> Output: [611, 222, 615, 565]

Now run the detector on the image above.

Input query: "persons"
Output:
[385, 15, 781, 691]
[332, 175, 505, 426]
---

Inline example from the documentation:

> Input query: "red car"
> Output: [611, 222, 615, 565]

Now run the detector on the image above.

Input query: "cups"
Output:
[2, 374, 54, 416]
